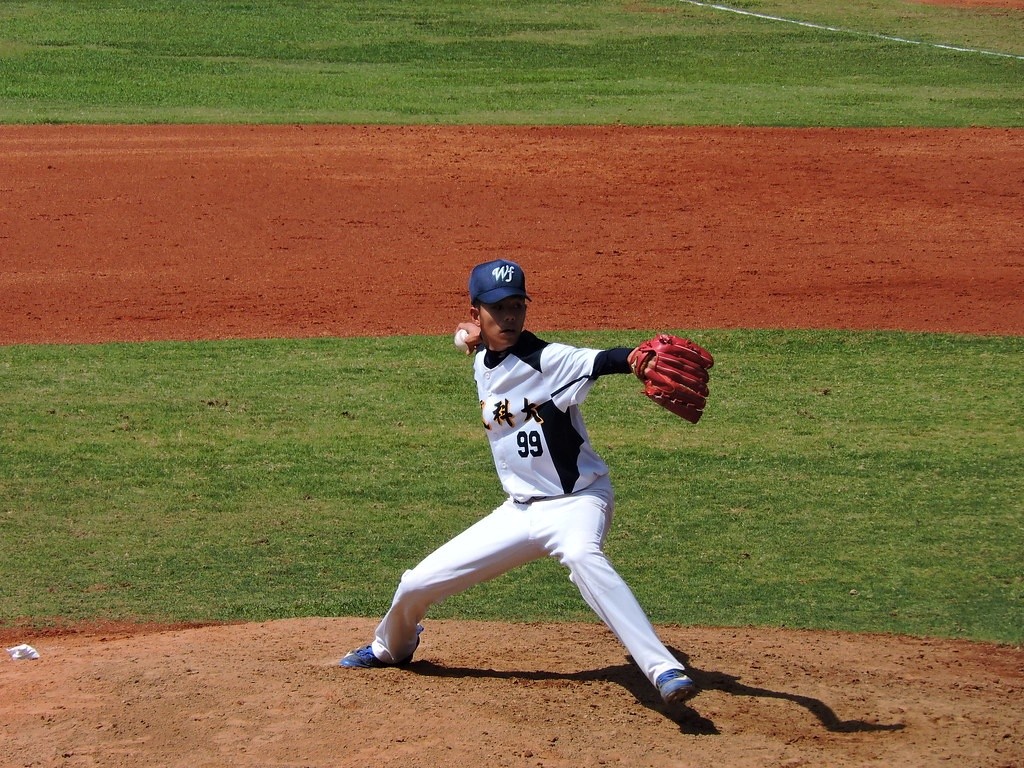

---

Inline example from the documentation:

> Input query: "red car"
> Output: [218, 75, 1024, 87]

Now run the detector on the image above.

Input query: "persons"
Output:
[339, 258, 717, 709]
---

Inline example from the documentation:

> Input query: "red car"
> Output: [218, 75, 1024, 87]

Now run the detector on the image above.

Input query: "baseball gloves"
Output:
[629, 333, 713, 425]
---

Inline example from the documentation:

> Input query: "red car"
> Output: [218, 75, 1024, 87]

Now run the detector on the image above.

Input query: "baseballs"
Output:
[453, 329, 469, 349]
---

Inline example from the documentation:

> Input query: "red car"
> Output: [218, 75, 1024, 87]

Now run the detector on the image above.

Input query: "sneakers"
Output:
[340, 625, 424, 667]
[656, 669, 696, 704]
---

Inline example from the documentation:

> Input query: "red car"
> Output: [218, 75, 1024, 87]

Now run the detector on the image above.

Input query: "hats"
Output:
[469, 259, 533, 305]
